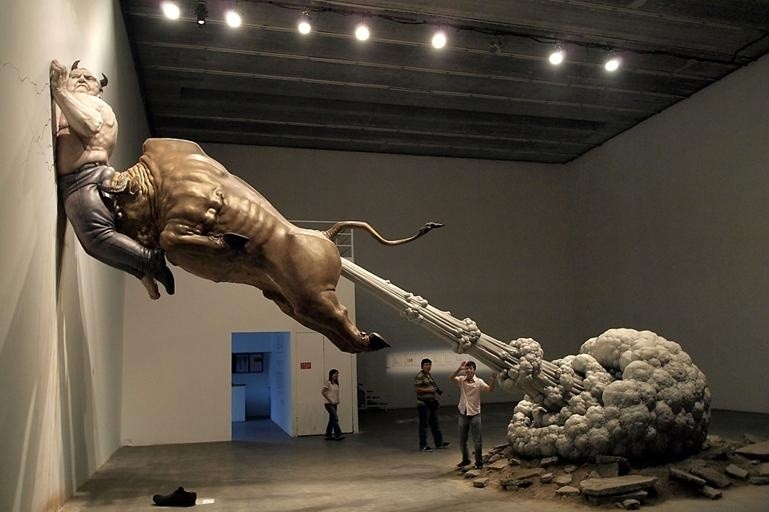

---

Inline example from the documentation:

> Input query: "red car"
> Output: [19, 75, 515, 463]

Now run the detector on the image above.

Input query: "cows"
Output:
[95, 136, 447, 354]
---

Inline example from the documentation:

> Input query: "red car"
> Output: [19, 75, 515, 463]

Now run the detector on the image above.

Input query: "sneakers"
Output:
[151, 486, 198, 507]
[336, 435, 346, 441]
[325, 435, 336, 441]
[419, 445, 433, 451]
[149, 247, 176, 295]
[474, 460, 483, 469]
[456, 459, 471, 467]
[437, 441, 451, 449]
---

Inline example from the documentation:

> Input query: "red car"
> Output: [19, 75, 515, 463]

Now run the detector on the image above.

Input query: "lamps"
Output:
[193, 3, 209, 30]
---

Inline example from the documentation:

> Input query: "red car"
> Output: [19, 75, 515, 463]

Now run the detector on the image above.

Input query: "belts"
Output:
[57, 159, 108, 183]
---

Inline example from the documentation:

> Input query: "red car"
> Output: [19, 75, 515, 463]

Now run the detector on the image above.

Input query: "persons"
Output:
[448, 360, 497, 470]
[414, 358, 451, 453]
[49, 58, 176, 301]
[319, 368, 346, 442]
